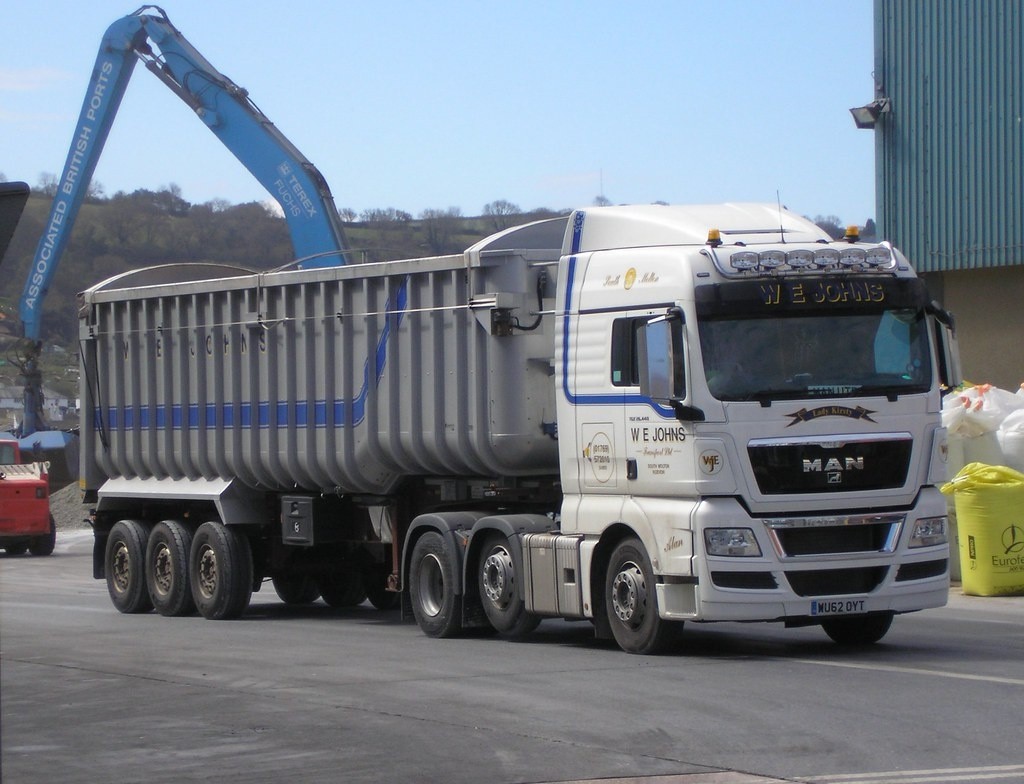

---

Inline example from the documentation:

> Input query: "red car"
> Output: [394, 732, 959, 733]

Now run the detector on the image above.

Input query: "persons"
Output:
[706, 335, 784, 401]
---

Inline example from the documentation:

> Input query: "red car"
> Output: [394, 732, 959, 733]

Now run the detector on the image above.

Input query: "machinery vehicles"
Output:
[0, 440, 55, 556]
[0, 5, 353, 483]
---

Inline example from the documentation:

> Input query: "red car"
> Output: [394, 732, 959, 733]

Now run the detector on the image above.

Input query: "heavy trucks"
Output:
[77, 201, 962, 656]
